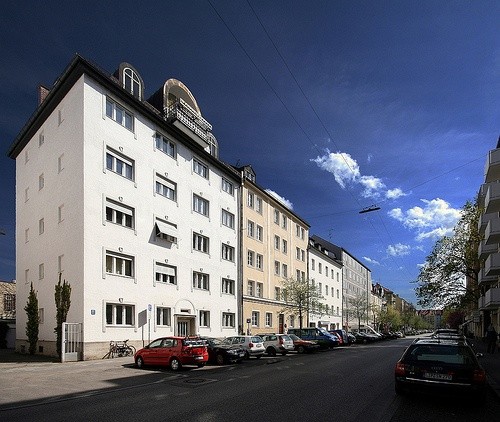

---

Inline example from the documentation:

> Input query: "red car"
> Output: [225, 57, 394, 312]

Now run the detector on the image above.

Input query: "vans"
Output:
[287, 327, 339, 352]
[351, 325, 382, 342]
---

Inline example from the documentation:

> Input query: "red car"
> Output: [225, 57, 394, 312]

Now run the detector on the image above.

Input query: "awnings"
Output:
[462, 320, 473, 325]
[156, 221, 182, 243]
[7, 324, 16, 328]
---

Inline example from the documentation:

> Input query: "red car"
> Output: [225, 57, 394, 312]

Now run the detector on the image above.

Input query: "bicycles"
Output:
[102, 339, 136, 360]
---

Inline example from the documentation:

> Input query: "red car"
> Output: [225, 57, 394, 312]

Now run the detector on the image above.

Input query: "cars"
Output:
[134, 335, 209, 372]
[393, 328, 488, 407]
[379, 332, 403, 340]
[223, 335, 266, 360]
[288, 334, 317, 353]
[354, 331, 377, 344]
[328, 332, 342, 347]
[200, 337, 247, 365]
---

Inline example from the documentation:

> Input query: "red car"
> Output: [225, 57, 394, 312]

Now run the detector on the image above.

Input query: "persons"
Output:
[485, 324, 497, 353]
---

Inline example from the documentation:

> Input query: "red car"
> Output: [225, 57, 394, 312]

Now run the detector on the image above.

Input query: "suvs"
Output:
[328, 329, 349, 346]
[255, 332, 295, 357]
